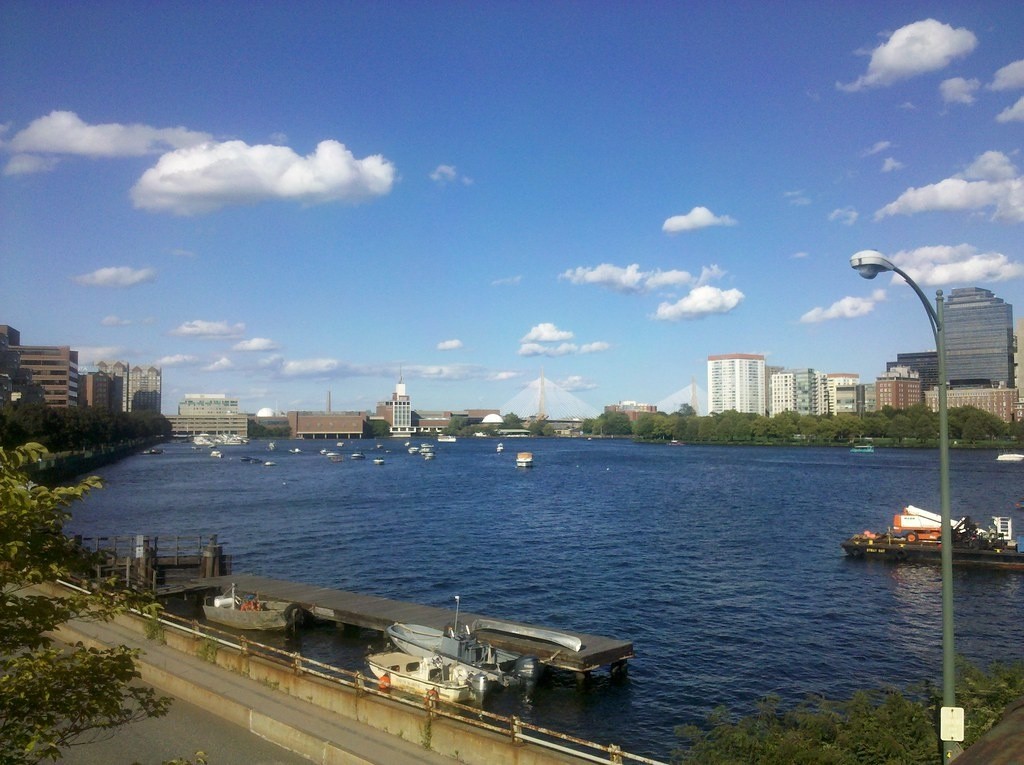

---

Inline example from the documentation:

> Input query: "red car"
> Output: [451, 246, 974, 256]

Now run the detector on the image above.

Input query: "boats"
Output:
[516, 452, 534, 468]
[201, 582, 305, 634]
[387, 596, 540, 691]
[140, 427, 506, 467]
[365, 648, 491, 703]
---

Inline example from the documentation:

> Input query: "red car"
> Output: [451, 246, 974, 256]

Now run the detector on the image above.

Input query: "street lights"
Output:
[849, 250, 965, 765]
